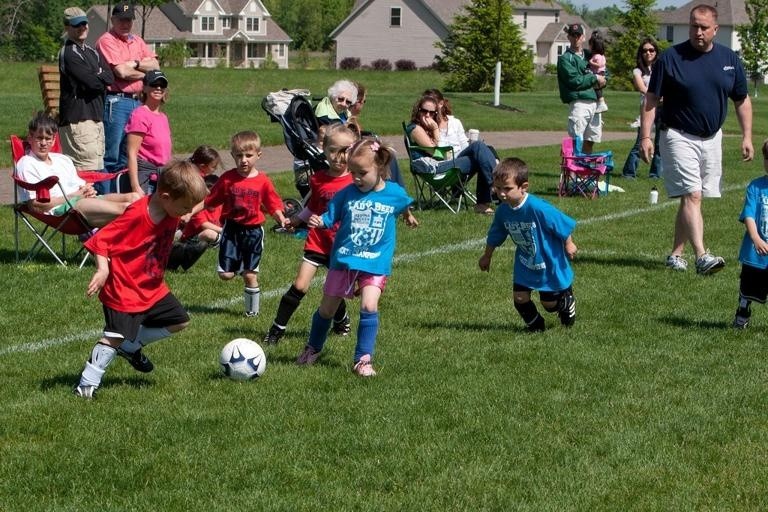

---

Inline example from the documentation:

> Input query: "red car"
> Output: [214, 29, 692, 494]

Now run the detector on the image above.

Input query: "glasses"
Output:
[33, 134, 52, 143]
[642, 48, 655, 53]
[419, 108, 436, 116]
[337, 96, 352, 106]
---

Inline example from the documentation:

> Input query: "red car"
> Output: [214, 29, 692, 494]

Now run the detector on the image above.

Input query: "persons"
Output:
[557, 23, 609, 157]
[295, 134, 420, 380]
[311, 79, 401, 185]
[16, 112, 142, 228]
[58, 4, 225, 275]
[477, 153, 582, 336]
[260, 117, 372, 348]
[642, 3, 753, 275]
[178, 128, 288, 318]
[72, 159, 210, 402]
[623, 36, 661, 179]
[407, 88, 501, 215]
[732, 140, 768, 329]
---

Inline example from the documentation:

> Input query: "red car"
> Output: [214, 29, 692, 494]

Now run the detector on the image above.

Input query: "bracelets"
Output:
[637, 135, 651, 140]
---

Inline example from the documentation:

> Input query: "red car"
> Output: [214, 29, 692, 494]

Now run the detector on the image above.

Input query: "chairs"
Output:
[9, 134, 133, 269]
[402, 121, 492, 214]
[557, 137, 606, 200]
[573, 135, 614, 197]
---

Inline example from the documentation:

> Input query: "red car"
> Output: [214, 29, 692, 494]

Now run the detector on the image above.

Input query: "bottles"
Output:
[648, 184, 659, 206]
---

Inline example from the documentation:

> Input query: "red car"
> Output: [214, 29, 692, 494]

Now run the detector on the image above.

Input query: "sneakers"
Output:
[245, 309, 376, 378]
[695, 252, 726, 275]
[117, 346, 153, 372]
[666, 255, 687, 270]
[595, 105, 608, 113]
[558, 294, 575, 327]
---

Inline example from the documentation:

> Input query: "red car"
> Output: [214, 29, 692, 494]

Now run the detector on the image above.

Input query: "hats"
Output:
[143, 70, 168, 88]
[112, 3, 136, 20]
[63, 6, 89, 26]
[569, 24, 583, 34]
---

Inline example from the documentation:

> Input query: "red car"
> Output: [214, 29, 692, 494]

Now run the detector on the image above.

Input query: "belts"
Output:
[107, 91, 139, 100]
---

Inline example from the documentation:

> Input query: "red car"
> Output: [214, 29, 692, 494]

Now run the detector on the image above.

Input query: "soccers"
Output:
[220, 338, 266, 380]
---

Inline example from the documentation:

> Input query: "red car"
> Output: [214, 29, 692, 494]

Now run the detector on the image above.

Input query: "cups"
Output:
[465, 128, 480, 146]
